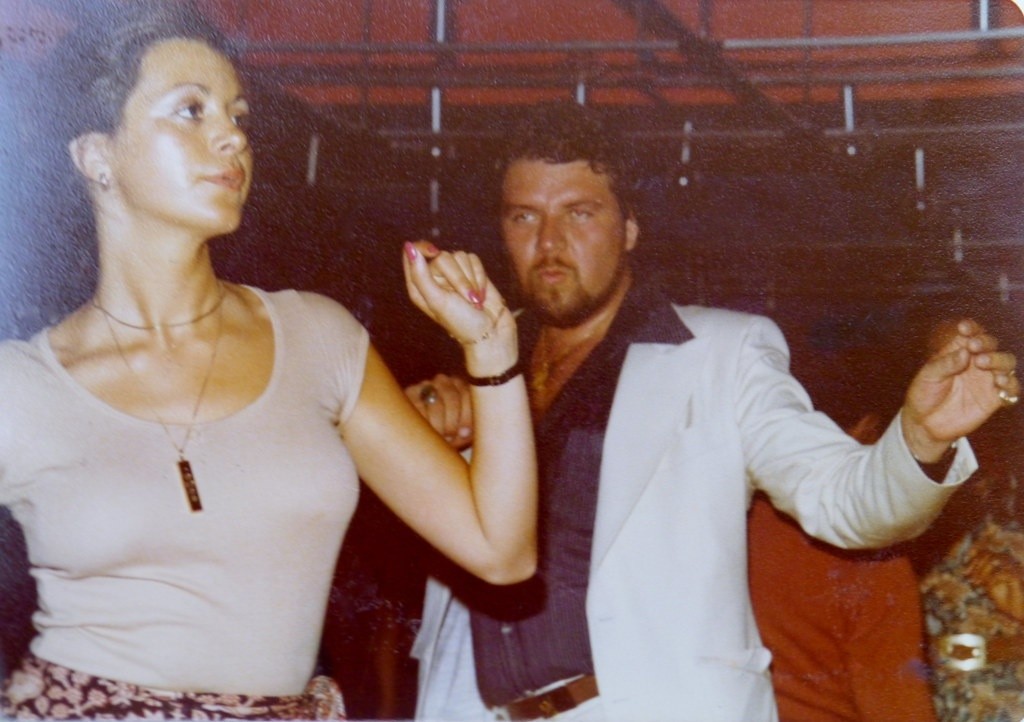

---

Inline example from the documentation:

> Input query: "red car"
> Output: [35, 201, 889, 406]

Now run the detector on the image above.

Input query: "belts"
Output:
[924, 632, 1023, 671]
[486, 673, 598, 721]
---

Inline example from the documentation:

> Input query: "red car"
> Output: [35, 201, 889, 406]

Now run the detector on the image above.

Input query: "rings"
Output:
[419, 385, 438, 403]
[997, 386, 1021, 408]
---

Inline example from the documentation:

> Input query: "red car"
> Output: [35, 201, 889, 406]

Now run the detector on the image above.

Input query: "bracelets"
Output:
[447, 298, 507, 345]
[462, 354, 526, 385]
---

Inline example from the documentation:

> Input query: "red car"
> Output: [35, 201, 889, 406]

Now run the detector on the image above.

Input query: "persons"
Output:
[0, 2, 538, 722]
[405, 102, 1024, 722]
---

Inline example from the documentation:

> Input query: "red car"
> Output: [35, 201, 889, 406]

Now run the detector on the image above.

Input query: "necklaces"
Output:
[529, 297, 622, 392]
[82, 281, 229, 510]
